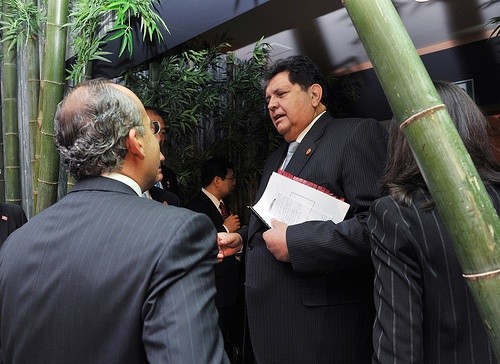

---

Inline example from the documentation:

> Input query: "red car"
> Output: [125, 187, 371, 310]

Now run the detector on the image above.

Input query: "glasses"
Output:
[133, 121, 161, 134]
[224, 175, 235, 181]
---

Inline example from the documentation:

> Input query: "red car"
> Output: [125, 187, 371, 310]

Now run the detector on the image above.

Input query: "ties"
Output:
[281, 141, 299, 172]
[219, 202, 229, 219]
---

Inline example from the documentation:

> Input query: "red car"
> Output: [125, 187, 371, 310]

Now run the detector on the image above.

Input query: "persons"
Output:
[0, 166, 28, 249]
[217, 55, 389, 364]
[366, 80, 500, 364]
[0, 79, 231, 364]
[144, 106, 181, 206]
[185, 155, 241, 364]
